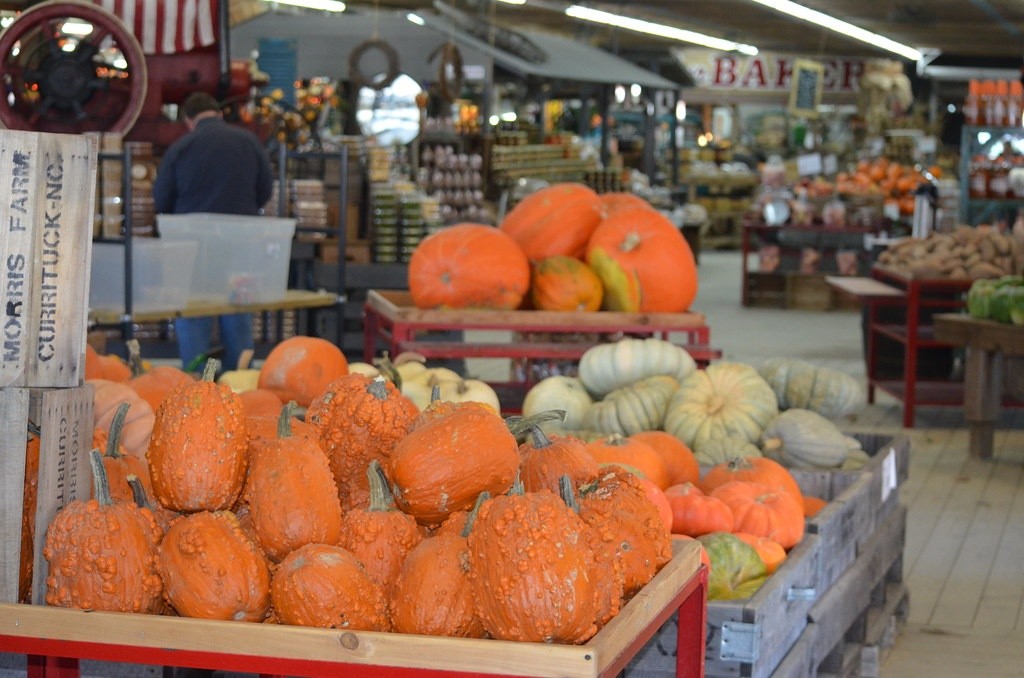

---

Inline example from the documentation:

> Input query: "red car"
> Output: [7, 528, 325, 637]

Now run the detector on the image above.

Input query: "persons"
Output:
[153, 94, 274, 384]
[998, 134, 1022, 158]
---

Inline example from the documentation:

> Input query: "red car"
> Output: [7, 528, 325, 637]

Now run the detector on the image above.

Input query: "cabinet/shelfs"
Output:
[87, 120, 515, 379]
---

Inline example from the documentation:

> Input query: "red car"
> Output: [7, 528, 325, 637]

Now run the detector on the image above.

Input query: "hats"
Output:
[182, 91, 221, 120]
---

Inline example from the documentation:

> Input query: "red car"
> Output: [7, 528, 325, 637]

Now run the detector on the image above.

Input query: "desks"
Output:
[825, 267, 1024, 430]
[739, 215, 911, 306]
[364, 289, 723, 425]
[0, 531, 709, 678]
[930, 313, 1024, 461]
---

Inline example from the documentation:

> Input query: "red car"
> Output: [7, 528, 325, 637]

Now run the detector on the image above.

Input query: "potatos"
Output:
[876, 224, 1024, 279]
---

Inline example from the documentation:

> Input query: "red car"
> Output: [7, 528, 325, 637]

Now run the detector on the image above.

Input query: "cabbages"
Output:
[966, 274, 1024, 327]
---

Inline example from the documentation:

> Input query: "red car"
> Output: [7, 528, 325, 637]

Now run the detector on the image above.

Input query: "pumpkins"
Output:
[17, 337, 872, 647]
[410, 182, 699, 314]
[827, 155, 943, 215]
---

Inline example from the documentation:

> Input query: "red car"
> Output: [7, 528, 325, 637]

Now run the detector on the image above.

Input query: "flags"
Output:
[92, 0, 217, 55]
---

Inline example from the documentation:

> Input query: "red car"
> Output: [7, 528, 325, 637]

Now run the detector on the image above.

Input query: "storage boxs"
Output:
[704, 463, 880, 678]
[835, 423, 911, 647]
[88, 236, 197, 313]
[621, 531, 825, 677]
[784, 271, 831, 310]
[157, 213, 298, 301]
[0, 127, 100, 387]
[0, 381, 95, 607]
